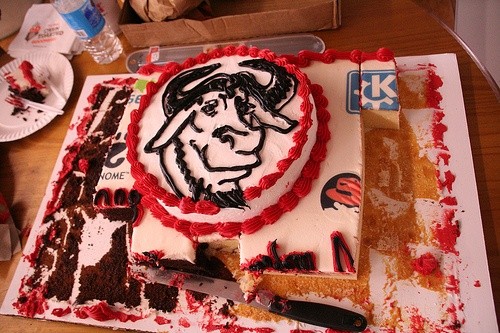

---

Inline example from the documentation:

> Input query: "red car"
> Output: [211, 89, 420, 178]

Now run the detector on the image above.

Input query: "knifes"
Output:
[145, 268, 367, 331]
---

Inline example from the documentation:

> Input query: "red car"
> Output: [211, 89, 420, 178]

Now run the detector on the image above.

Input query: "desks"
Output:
[0, 0, 500, 333]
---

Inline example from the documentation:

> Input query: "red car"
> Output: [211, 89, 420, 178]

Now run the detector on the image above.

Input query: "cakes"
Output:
[92, 47, 400, 303]
[4, 60, 49, 105]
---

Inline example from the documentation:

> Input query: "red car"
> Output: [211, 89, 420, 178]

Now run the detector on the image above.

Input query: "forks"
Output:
[32, 64, 67, 104]
[5, 96, 65, 115]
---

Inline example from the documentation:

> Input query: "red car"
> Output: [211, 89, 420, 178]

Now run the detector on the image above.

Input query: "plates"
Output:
[0, 50, 74, 142]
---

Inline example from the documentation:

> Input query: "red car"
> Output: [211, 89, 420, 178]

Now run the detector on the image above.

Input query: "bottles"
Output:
[50, 0, 124, 65]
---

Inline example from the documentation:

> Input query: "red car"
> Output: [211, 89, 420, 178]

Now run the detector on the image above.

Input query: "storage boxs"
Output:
[117, 0, 343, 49]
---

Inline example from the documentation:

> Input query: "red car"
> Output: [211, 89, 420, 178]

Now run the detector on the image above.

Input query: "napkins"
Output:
[7, 4, 84, 60]
